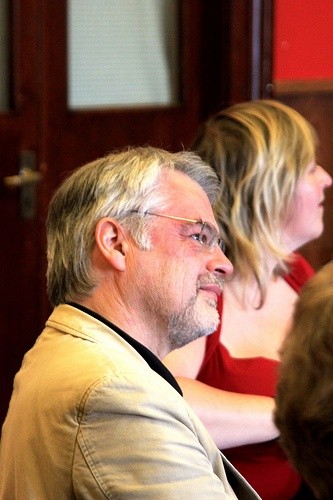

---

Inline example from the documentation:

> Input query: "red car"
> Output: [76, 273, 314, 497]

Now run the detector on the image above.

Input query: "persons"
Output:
[0, 141, 262, 500]
[272, 260, 333, 500]
[161, 99, 332, 500]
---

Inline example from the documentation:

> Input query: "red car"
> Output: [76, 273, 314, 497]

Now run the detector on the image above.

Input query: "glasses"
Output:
[92, 209, 225, 253]
